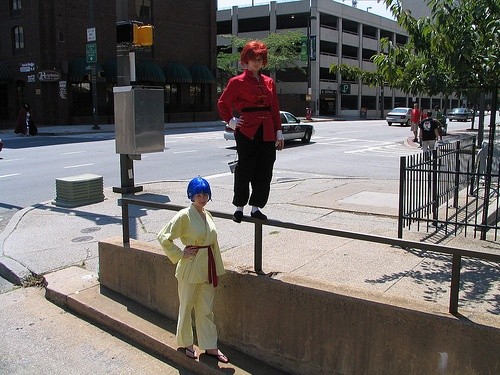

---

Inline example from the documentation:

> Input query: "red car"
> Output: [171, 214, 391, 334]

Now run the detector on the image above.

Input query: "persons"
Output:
[217, 40, 284, 223]
[158, 177, 229, 363]
[410, 103, 421, 142]
[418, 107, 427, 148]
[434, 105, 444, 143]
[14, 103, 38, 136]
[419, 110, 438, 163]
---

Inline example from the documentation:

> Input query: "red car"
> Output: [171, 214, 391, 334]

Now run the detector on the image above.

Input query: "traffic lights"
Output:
[114, 19, 139, 47]
[83, 73, 91, 81]
[139, 21, 156, 48]
[98, 71, 105, 79]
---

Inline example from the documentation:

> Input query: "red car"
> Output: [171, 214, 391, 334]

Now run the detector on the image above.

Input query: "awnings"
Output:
[68, 59, 103, 79]
[163, 63, 193, 84]
[190, 63, 215, 84]
[104, 58, 165, 85]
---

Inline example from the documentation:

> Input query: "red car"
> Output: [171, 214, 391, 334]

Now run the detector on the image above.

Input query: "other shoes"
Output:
[251, 210, 268, 221]
[233, 210, 244, 224]
[205, 350, 229, 363]
[185, 347, 197, 358]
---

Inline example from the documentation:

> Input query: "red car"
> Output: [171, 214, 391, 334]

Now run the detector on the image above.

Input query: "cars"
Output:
[385, 107, 413, 127]
[224, 110, 314, 144]
[447, 107, 474, 122]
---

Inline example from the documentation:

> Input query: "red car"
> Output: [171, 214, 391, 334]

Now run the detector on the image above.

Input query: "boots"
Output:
[26, 128, 30, 137]
